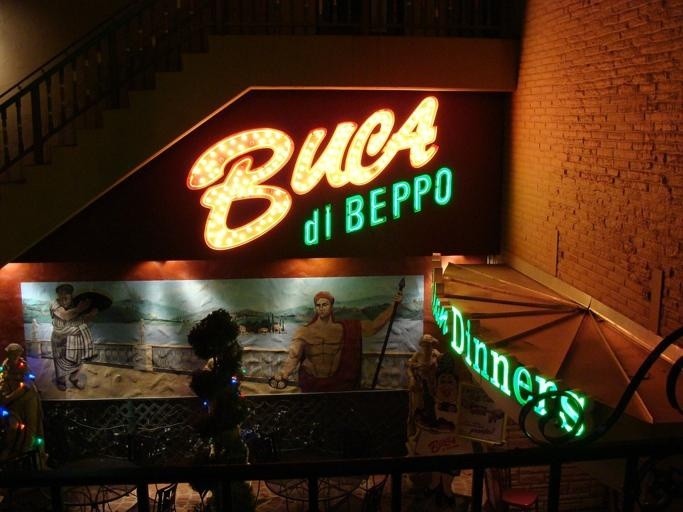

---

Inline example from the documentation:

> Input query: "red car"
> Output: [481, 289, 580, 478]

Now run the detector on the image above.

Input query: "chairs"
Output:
[334, 476, 389, 509]
[130, 483, 177, 511]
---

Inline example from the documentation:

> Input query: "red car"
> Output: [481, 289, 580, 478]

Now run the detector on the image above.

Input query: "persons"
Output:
[49, 284, 98, 391]
[406, 334, 445, 434]
[1, 342, 40, 452]
[274, 290, 404, 393]
